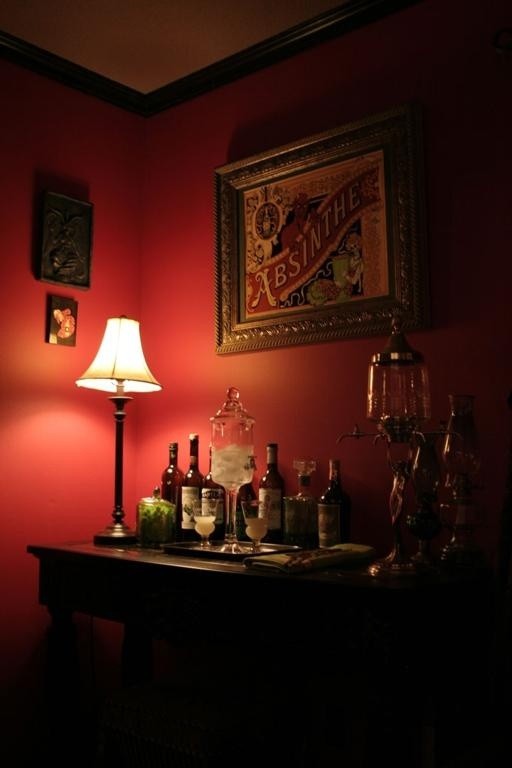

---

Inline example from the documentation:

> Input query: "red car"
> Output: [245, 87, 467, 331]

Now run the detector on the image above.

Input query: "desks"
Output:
[27, 542, 493, 768]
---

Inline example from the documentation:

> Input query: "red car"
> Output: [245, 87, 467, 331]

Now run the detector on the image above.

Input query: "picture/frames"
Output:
[213, 105, 420, 357]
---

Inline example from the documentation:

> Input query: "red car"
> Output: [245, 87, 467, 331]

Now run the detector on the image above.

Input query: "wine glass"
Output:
[332, 255, 350, 302]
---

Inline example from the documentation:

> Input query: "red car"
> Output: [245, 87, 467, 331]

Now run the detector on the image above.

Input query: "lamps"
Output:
[74, 315, 163, 547]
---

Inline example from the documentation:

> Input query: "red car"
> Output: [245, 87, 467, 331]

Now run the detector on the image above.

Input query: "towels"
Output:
[242, 542, 377, 576]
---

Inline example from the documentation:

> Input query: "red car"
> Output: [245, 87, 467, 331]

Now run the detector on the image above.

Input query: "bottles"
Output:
[134, 386, 349, 555]
[260, 206, 272, 237]
